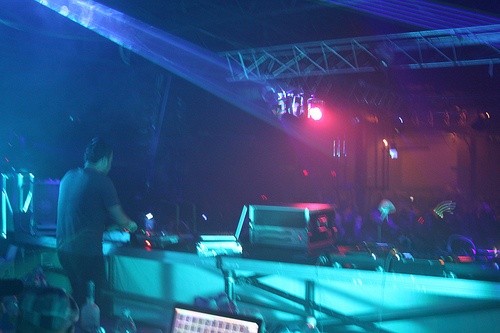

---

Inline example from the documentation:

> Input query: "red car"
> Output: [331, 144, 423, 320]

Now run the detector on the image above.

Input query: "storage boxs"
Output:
[248, 202, 337, 252]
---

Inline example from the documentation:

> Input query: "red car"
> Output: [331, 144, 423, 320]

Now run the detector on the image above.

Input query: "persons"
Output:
[56, 135, 138, 333]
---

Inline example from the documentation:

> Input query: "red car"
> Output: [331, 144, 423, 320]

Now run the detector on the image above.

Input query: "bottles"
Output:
[80, 280, 100, 333]
[302, 317, 320, 333]
[115, 309, 137, 333]
